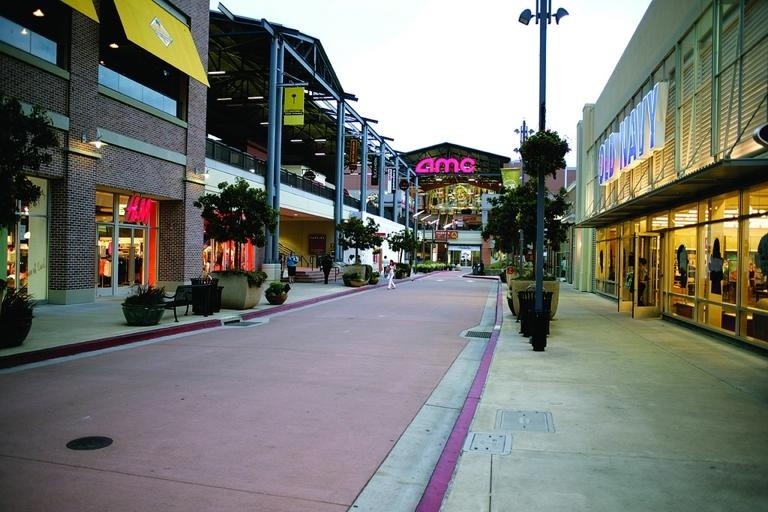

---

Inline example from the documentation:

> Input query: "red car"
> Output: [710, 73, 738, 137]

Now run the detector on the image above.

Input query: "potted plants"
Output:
[483, 183, 568, 319]
[350, 273, 364, 287]
[369, 271, 380, 285]
[336, 217, 384, 280]
[0, 283, 39, 349]
[342, 272, 352, 285]
[192, 176, 280, 310]
[121, 287, 167, 325]
[264, 282, 291, 305]
[413, 263, 452, 273]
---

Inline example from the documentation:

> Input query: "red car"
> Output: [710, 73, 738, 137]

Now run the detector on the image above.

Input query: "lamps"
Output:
[82, 126, 108, 149]
[195, 162, 211, 179]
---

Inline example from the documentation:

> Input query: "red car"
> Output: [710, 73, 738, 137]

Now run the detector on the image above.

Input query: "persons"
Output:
[286, 250, 299, 283]
[317, 253, 323, 271]
[358, 255, 361, 263]
[472, 260, 480, 274]
[630, 256, 650, 307]
[386, 259, 397, 290]
[348, 254, 354, 264]
[560, 255, 567, 277]
[382, 255, 389, 275]
[478, 259, 486, 275]
[408, 256, 413, 267]
[320, 252, 333, 284]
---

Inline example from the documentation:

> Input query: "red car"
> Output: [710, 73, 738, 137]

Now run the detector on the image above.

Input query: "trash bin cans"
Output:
[517, 291, 554, 338]
[190, 278, 224, 317]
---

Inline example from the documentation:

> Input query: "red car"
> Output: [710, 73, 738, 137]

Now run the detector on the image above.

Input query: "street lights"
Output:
[518, 0, 570, 351]
[513, 120, 535, 277]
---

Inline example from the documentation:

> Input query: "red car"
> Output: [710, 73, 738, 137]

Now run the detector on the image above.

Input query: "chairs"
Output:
[163, 285, 193, 322]
